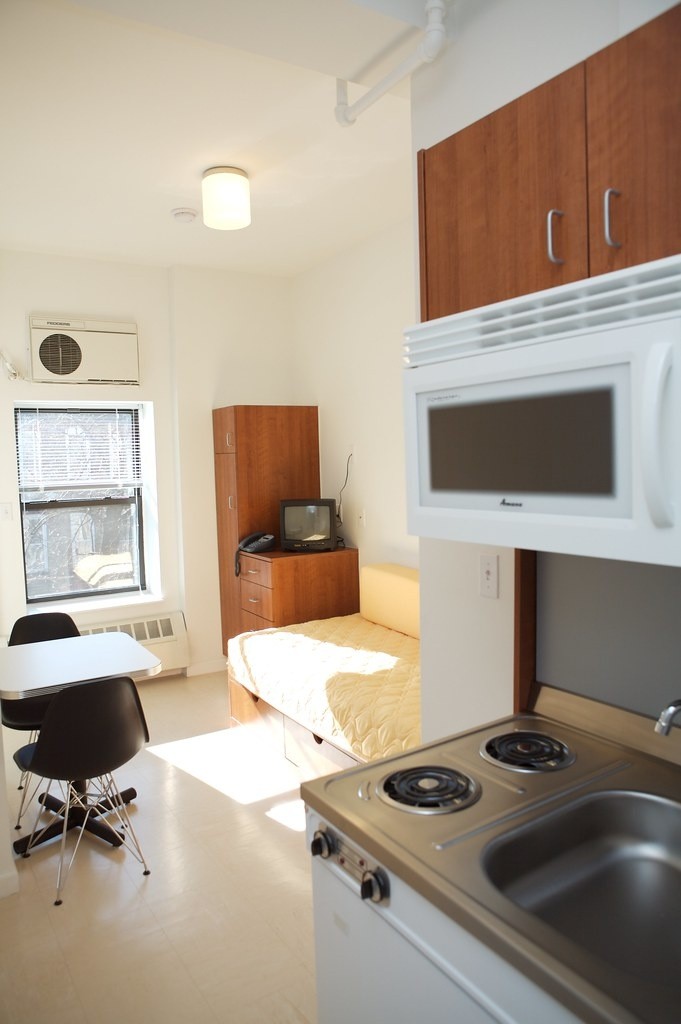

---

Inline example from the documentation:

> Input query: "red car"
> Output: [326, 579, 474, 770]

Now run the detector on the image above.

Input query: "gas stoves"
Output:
[300, 713, 636, 904]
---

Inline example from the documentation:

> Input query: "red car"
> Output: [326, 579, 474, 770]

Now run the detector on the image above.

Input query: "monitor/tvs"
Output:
[279, 498, 338, 552]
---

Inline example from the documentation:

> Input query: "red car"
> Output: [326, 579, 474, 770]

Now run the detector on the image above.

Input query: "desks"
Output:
[0, 631, 163, 859]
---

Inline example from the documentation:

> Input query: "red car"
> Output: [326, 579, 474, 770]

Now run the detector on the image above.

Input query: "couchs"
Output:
[232, 563, 420, 762]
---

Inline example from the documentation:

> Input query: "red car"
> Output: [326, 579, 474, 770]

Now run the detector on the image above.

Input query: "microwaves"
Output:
[403, 253, 680, 567]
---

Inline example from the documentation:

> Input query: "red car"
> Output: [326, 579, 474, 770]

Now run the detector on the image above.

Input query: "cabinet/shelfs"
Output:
[415, 0, 681, 325]
[212, 405, 322, 656]
[225, 662, 357, 739]
[238, 545, 360, 634]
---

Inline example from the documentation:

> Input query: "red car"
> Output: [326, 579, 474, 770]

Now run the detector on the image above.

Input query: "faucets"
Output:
[654, 700, 681, 737]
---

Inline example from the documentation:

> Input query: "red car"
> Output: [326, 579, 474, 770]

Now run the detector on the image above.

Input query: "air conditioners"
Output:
[28, 314, 141, 386]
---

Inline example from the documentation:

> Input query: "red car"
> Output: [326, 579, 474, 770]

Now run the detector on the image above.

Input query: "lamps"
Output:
[202, 166, 251, 230]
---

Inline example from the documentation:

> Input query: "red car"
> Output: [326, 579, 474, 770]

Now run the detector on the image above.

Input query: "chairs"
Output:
[0, 611, 127, 835]
[13, 675, 152, 908]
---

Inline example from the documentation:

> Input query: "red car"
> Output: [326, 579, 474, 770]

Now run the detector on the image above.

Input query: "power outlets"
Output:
[336, 504, 344, 522]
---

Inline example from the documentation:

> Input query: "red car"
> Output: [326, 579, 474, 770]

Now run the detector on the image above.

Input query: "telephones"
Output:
[239, 531, 277, 554]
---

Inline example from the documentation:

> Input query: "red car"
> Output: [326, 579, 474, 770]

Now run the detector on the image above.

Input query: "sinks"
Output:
[436, 759, 680, 1024]
